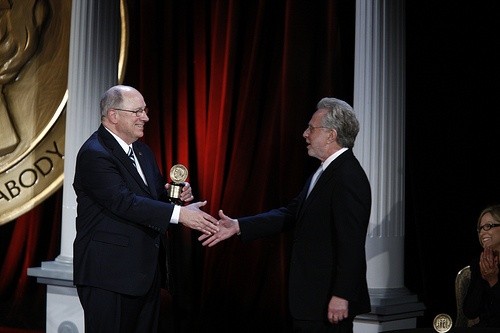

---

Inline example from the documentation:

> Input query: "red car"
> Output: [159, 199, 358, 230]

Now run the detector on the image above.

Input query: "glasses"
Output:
[310, 126, 327, 133]
[478, 223, 500, 232]
[115, 107, 147, 117]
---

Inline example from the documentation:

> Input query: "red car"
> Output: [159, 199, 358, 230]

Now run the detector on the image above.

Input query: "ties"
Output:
[127, 147, 136, 167]
[306, 163, 324, 197]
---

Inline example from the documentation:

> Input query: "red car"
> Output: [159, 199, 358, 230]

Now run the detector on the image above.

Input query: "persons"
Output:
[73, 84, 219, 333]
[198, 97, 371, 333]
[449, 206, 500, 333]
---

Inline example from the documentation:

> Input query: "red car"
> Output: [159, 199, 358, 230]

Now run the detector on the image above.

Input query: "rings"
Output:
[485, 271, 487, 272]
